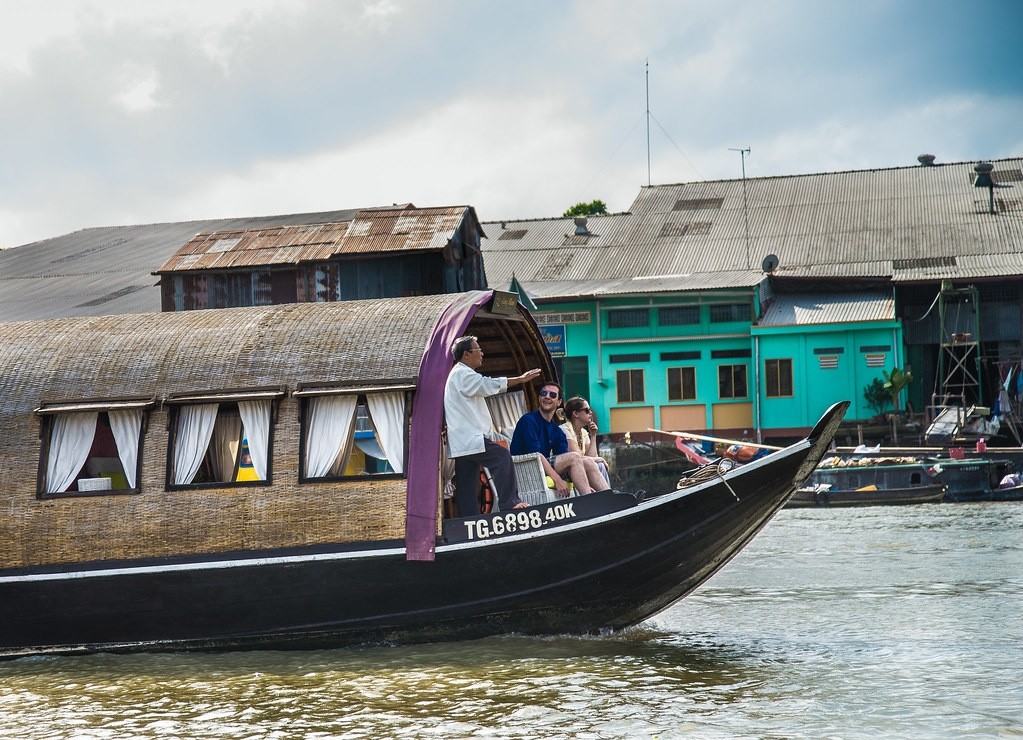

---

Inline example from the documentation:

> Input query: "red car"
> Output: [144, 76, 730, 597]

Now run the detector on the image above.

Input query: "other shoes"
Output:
[635, 489, 646, 502]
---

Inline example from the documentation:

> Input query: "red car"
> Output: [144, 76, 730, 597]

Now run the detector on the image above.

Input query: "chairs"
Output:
[87, 444, 396, 492]
[481, 431, 610, 514]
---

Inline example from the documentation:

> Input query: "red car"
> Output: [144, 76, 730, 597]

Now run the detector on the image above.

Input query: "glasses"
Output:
[539, 391, 559, 400]
[576, 407, 591, 414]
[468, 349, 482, 352]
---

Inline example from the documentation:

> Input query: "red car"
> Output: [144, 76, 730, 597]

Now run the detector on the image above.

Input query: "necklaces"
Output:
[573, 423, 582, 450]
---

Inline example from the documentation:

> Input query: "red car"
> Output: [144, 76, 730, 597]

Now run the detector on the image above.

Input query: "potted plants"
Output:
[864, 378, 892, 425]
[874, 369, 913, 424]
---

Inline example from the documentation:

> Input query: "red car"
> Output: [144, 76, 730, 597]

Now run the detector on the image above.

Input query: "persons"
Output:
[510, 381, 621, 496]
[556, 396, 646, 502]
[444, 336, 541, 518]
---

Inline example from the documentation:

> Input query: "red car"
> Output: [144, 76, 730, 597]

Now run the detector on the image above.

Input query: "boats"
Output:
[0, 288, 855, 664]
[801, 456, 1023, 504]
[780, 482, 949, 510]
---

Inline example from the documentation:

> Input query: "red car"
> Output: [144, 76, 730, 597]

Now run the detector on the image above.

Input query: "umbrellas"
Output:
[509, 271, 538, 313]
